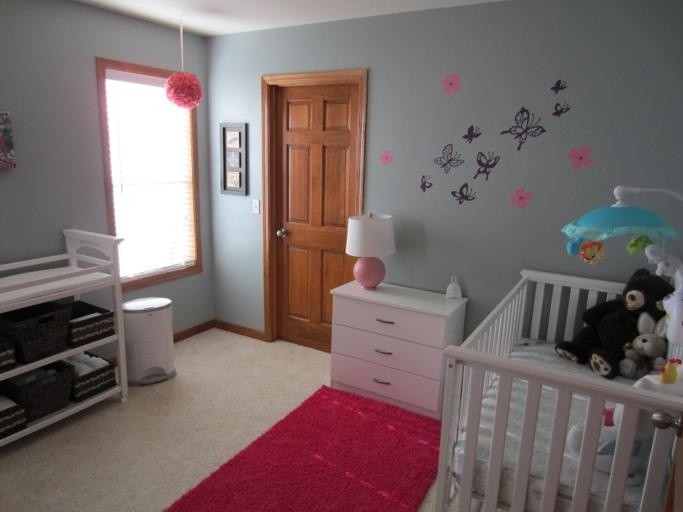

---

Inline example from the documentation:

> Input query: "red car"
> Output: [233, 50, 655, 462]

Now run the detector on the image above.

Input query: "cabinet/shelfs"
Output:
[0, 229, 133, 447]
[330, 279, 470, 420]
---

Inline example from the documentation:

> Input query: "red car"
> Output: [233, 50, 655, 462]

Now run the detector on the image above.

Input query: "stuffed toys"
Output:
[567, 365, 683, 484]
[556, 244, 683, 381]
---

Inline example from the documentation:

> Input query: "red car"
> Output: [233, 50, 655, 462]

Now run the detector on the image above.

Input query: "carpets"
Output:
[161, 385, 441, 512]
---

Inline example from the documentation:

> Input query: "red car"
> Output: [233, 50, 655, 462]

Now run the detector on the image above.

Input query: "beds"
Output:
[435, 267, 683, 512]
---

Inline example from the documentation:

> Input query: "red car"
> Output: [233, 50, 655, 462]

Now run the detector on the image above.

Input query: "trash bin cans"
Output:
[122, 296, 176, 386]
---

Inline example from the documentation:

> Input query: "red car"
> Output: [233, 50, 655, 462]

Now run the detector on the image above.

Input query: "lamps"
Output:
[345, 211, 397, 290]
[164, 24, 203, 109]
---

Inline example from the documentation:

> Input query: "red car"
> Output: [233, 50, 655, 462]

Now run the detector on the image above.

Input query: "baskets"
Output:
[0, 301, 71, 365]
[0, 394, 28, 440]
[70, 351, 116, 403]
[68, 299, 115, 349]
[0, 360, 75, 424]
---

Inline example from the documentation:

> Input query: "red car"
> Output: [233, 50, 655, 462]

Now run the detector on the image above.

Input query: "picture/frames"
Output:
[219, 122, 248, 196]
